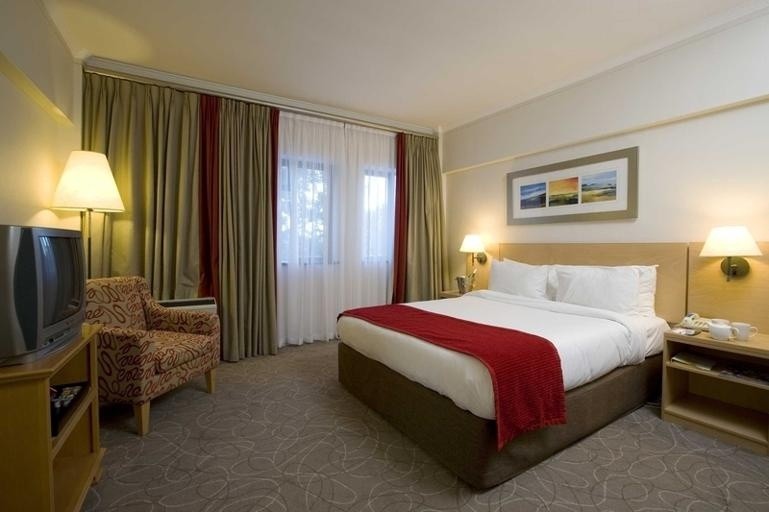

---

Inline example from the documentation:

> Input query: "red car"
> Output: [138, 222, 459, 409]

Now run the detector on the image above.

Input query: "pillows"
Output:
[488, 257, 660, 319]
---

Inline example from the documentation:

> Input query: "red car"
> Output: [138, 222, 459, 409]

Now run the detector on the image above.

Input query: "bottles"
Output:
[468, 268, 478, 279]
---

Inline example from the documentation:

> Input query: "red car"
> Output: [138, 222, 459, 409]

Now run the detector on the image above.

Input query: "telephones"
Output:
[680, 313, 712, 332]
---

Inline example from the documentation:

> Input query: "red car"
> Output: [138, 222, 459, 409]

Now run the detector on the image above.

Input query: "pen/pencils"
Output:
[469, 269, 478, 278]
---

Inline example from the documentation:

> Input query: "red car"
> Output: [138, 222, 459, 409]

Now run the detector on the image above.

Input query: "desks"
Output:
[1, 322, 105, 511]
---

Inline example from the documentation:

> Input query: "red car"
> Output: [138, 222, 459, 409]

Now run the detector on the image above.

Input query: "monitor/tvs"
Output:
[0, 224, 87, 368]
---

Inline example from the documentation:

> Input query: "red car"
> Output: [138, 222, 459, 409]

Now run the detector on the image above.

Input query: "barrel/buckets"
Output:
[456, 276, 474, 294]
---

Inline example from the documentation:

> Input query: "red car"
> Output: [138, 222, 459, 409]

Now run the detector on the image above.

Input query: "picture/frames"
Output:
[506, 146, 639, 225]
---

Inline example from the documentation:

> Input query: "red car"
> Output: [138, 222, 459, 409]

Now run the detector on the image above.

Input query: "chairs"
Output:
[84, 275, 221, 436]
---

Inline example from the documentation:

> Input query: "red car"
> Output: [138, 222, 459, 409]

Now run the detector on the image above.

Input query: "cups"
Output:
[732, 321, 758, 340]
[706, 318, 739, 340]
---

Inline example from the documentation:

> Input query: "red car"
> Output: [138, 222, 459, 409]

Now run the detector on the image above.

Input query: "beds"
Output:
[337, 243, 689, 490]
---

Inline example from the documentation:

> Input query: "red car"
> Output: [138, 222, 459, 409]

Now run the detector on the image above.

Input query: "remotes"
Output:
[689, 328, 701, 337]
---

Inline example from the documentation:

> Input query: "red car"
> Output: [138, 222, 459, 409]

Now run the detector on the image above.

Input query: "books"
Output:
[670, 349, 721, 372]
[719, 359, 769, 386]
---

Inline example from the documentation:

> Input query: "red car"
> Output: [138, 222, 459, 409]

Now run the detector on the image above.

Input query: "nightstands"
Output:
[661, 325, 769, 457]
[441, 290, 461, 299]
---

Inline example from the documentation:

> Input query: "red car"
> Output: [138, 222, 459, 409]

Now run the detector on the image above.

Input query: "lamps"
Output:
[460, 234, 487, 265]
[700, 225, 763, 282]
[49, 150, 125, 246]
